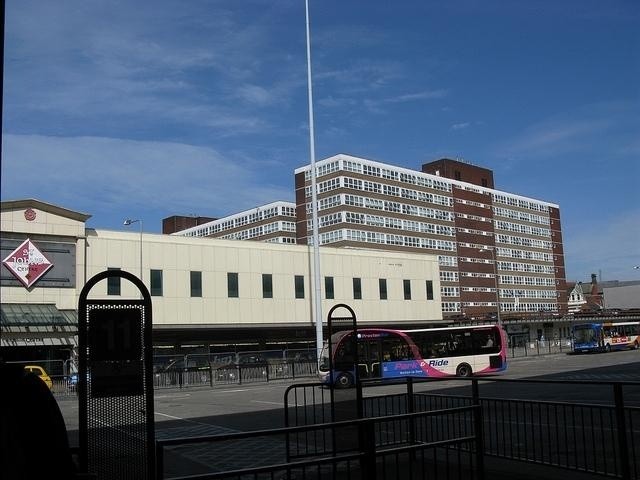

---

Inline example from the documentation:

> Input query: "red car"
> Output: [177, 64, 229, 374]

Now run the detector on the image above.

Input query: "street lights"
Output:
[124, 219, 142, 300]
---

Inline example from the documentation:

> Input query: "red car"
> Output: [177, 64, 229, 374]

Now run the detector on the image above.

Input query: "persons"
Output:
[386, 335, 465, 363]
[604, 326, 639, 337]
[480, 335, 494, 348]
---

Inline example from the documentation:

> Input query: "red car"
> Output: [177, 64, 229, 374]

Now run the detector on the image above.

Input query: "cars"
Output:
[156, 354, 267, 386]
[67, 367, 91, 391]
[24, 365, 53, 390]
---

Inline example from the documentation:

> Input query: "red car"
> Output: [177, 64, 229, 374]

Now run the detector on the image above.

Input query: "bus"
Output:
[573, 321, 640, 353]
[318, 324, 508, 389]
